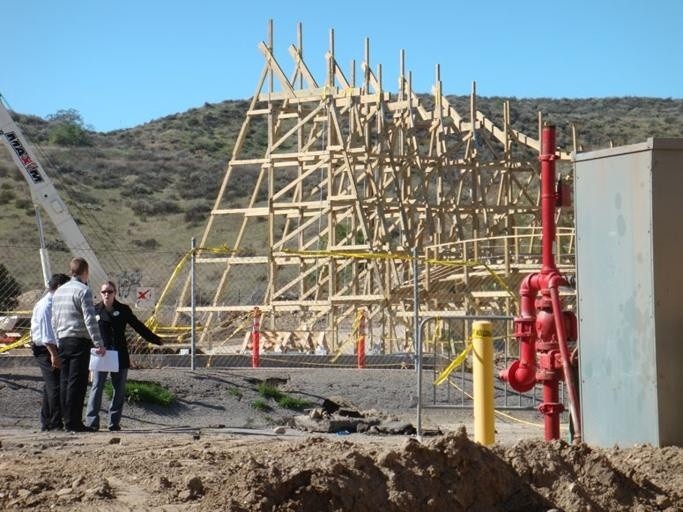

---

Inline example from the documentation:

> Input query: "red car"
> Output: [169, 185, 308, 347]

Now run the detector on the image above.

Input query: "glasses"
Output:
[101, 290, 113, 294]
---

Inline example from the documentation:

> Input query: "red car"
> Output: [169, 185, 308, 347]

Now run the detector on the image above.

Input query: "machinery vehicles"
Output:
[0, 95, 154, 346]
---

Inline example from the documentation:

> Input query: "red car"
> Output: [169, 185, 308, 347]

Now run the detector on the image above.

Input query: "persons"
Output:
[86, 281, 163, 431]
[30, 273, 70, 431]
[51, 256, 106, 432]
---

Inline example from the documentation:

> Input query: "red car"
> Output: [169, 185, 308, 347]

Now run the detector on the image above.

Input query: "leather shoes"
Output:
[42, 425, 93, 432]
